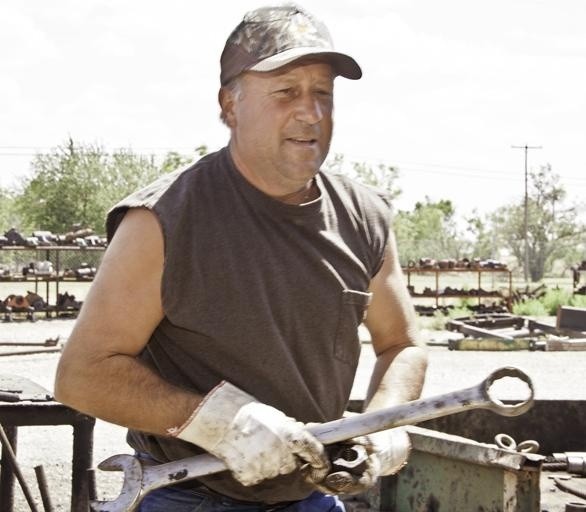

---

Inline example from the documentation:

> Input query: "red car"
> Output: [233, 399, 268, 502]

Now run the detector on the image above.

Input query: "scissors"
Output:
[495, 433, 540, 453]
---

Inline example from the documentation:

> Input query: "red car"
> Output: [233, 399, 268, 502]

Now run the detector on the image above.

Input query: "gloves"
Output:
[174, 380, 328, 486]
[299, 422, 411, 495]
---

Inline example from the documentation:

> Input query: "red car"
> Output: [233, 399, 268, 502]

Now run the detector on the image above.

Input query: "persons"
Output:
[52, 6, 429, 511]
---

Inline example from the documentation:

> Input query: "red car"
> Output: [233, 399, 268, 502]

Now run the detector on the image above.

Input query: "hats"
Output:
[220, 4, 361, 86]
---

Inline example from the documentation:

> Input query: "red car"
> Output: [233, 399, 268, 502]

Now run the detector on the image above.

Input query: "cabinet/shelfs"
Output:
[0, 235, 111, 350]
[401, 258, 514, 319]
